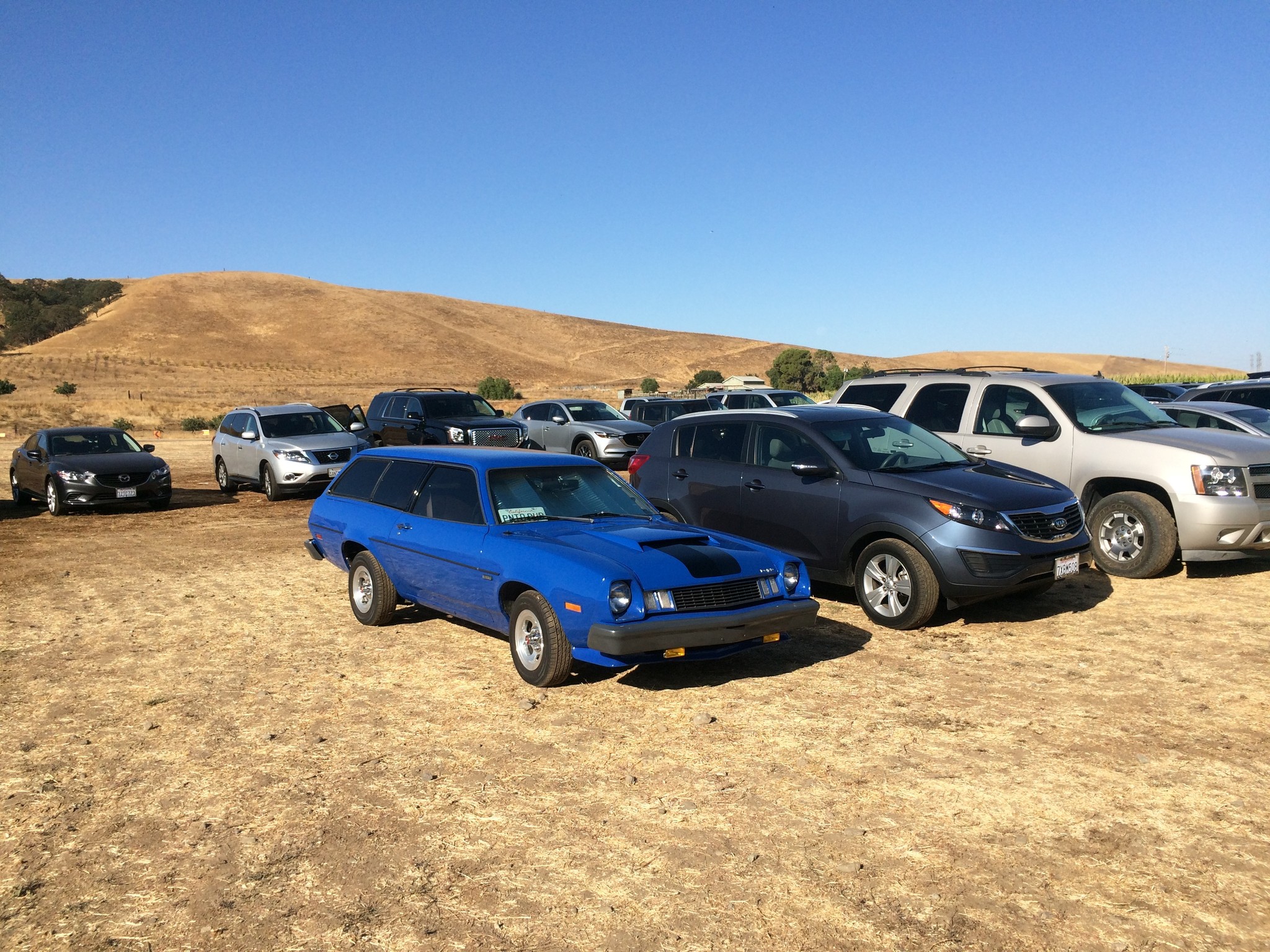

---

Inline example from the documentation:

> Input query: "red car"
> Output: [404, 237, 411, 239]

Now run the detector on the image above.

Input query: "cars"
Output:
[9, 426, 173, 516]
[1076, 371, 1270, 436]
[511, 399, 654, 464]
[620, 388, 832, 427]
[302, 443, 822, 687]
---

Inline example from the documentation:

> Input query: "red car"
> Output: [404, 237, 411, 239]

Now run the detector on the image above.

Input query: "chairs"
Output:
[984, 399, 1014, 434]
[98, 434, 112, 447]
[298, 417, 313, 430]
[768, 433, 800, 469]
[816, 424, 851, 463]
[52, 437, 65, 453]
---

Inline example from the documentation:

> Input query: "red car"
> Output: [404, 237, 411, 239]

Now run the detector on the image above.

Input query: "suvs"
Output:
[826, 364, 1270, 580]
[365, 387, 532, 448]
[626, 403, 1098, 632]
[211, 401, 374, 503]
